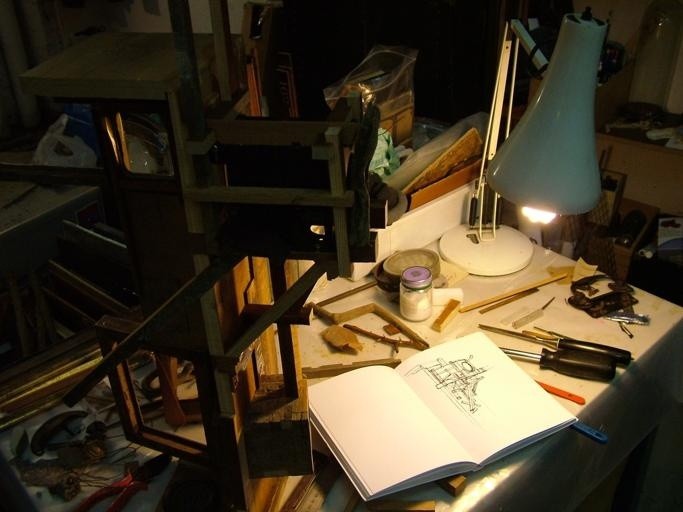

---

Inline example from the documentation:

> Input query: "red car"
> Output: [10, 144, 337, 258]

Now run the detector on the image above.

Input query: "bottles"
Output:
[398, 264, 433, 324]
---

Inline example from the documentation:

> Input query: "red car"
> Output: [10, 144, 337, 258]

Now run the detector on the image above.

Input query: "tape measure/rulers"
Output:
[458, 273, 567, 315]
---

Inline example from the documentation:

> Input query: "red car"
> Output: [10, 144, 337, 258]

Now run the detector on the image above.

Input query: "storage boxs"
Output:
[587, 167, 628, 227]
[584, 197, 660, 282]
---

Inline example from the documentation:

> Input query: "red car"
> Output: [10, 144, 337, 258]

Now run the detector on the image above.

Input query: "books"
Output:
[308, 329, 582, 501]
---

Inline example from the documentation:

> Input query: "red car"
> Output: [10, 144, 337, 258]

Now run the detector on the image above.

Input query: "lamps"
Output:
[437, 15, 601, 278]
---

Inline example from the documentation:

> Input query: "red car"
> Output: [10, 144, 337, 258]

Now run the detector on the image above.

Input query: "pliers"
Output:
[75, 453, 172, 512]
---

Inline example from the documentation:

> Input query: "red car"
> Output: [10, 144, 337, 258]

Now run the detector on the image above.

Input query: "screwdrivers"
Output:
[477, 327, 630, 381]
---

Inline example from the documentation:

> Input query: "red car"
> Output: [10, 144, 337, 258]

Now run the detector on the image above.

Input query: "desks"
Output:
[1, 240, 683, 512]
